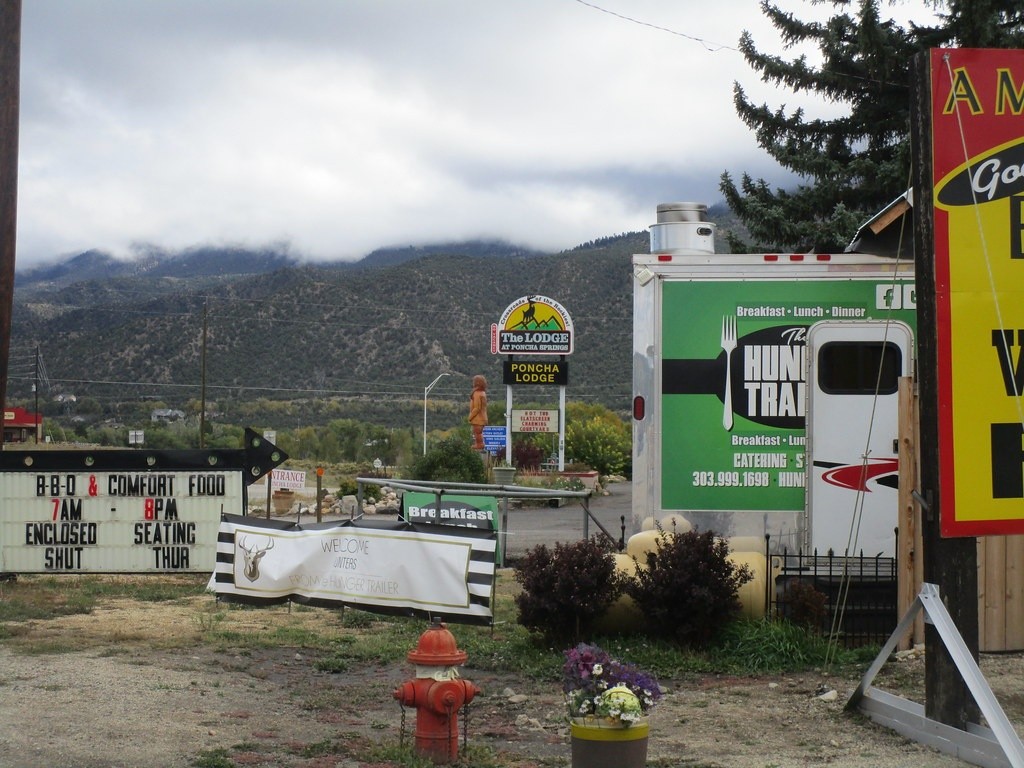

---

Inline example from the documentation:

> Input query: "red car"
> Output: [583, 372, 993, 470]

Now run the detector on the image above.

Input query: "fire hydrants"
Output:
[392, 617, 479, 765]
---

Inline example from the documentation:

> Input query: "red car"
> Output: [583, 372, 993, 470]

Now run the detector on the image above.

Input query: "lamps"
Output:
[636, 268, 655, 287]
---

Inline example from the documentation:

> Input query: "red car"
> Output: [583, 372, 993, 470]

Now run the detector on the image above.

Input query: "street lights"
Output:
[425, 372, 450, 458]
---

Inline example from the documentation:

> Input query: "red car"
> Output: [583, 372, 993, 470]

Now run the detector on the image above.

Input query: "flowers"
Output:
[562, 642, 663, 731]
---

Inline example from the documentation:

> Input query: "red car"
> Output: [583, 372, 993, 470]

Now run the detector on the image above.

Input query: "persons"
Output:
[468, 376, 488, 449]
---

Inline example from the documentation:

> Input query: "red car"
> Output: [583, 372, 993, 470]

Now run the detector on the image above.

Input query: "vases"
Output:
[569, 718, 650, 768]
[272, 490, 295, 514]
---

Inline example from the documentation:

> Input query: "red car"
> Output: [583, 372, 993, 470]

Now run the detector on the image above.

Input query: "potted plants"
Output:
[493, 459, 516, 486]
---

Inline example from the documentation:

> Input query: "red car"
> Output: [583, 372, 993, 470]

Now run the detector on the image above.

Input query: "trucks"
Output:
[633, 200, 916, 599]
